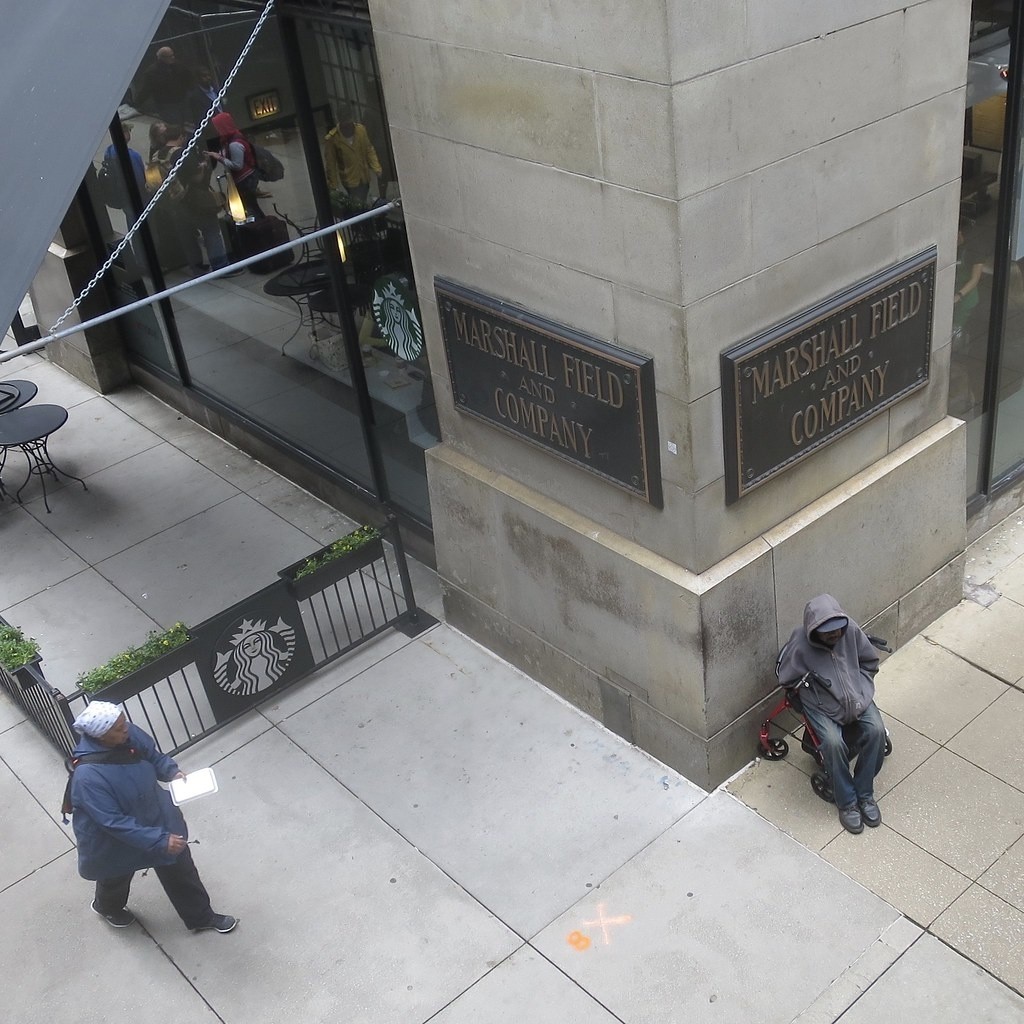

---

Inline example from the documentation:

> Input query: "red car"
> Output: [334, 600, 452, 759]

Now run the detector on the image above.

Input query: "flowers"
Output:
[80, 621, 190, 697]
[0, 624, 43, 671]
[295, 526, 377, 579]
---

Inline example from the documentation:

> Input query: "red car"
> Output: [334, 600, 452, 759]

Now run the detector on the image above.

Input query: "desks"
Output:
[264, 204, 363, 358]
[0, 380, 88, 513]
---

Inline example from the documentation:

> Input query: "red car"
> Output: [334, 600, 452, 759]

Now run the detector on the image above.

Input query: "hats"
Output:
[815, 618, 848, 633]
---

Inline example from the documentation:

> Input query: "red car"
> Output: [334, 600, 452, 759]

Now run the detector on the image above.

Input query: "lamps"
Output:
[225, 173, 247, 227]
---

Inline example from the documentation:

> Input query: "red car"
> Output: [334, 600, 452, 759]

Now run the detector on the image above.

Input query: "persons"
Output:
[154, 47, 190, 140]
[72, 700, 237, 934]
[190, 66, 273, 197]
[780, 593, 886, 835]
[102, 122, 146, 260]
[362, 100, 398, 200]
[952, 214, 986, 329]
[146, 114, 245, 279]
[202, 113, 262, 219]
[325, 104, 382, 200]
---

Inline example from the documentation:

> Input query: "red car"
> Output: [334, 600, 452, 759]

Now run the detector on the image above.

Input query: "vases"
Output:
[74, 631, 202, 705]
[276, 525, 381, 601]
[0, 616, 44, 691]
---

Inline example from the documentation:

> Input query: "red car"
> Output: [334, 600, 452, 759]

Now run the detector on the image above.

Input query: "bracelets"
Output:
[957, 291, 963, 298]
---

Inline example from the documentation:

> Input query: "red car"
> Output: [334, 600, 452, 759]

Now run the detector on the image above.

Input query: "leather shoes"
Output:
[840, 804, 864, 834]
[858, 796, 880, 827]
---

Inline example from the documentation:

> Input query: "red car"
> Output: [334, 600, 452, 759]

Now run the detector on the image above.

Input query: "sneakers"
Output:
[91, 899, 136, 927]
[197, 912, 236, 932]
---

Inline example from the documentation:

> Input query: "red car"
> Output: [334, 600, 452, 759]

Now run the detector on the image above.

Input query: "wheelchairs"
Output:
[756, 629, 894, 808]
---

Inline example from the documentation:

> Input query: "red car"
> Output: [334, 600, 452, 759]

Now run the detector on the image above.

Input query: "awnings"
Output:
[0, 0, 400, 360]
